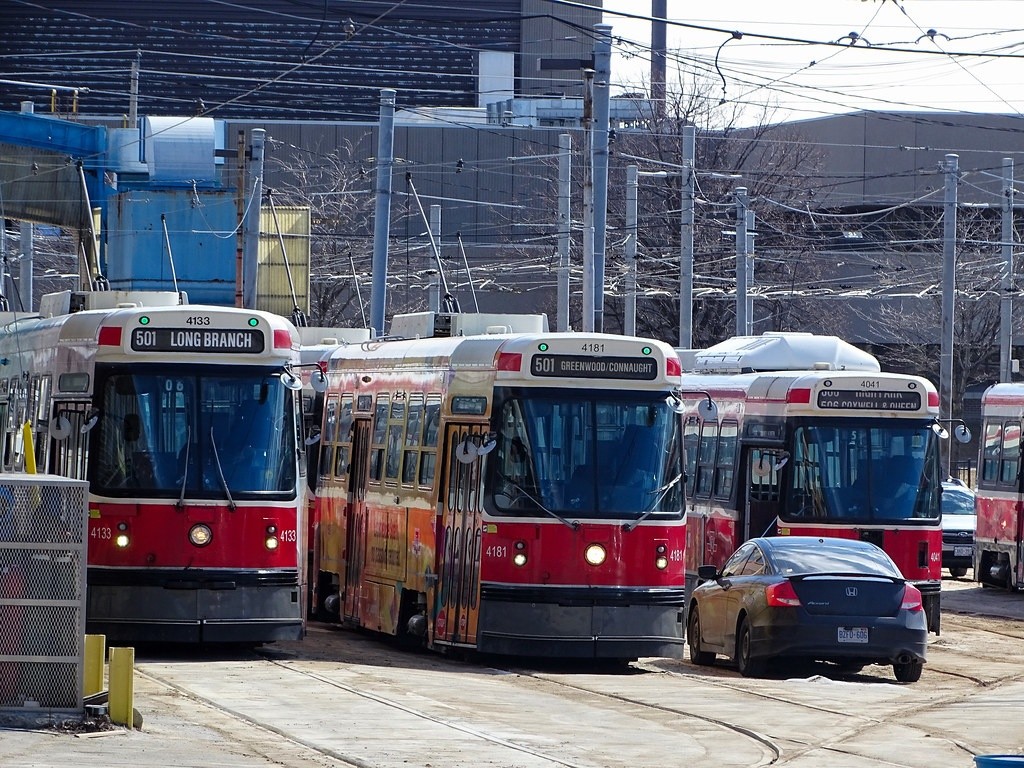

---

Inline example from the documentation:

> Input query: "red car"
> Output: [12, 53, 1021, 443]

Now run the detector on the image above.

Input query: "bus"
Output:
[667, 328, 943, 636]
[0, 206, 310, 653]
[296, 170, 695, 658]
[973, 384, 1024, 586]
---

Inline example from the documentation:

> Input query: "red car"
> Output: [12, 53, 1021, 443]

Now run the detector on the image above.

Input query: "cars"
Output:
[936, 478, 986, 573]
[684, 536, 931, 679]
